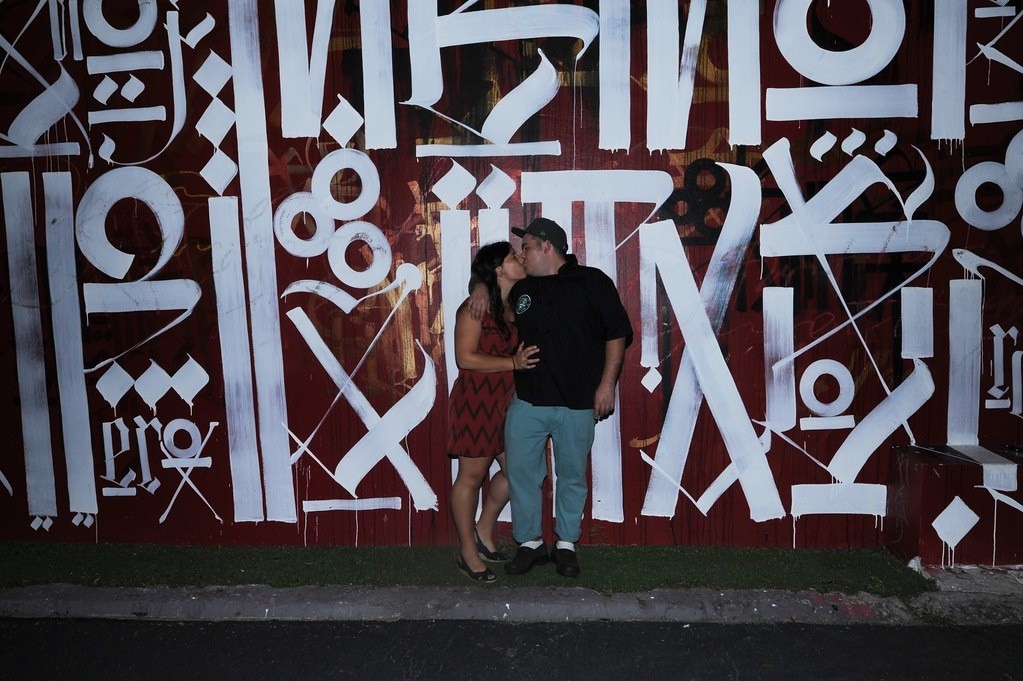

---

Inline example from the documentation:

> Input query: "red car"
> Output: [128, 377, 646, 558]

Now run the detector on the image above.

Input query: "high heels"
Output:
[474, 526, 510, 562]
[456, 552, 497, 583]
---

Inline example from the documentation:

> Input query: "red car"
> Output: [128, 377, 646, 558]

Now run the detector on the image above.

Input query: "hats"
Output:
[511, 218, 568, 252]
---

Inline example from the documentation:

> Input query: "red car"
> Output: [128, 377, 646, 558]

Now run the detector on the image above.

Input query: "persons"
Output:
[467, 218, 634, 577]
[448, 240, 541, 583]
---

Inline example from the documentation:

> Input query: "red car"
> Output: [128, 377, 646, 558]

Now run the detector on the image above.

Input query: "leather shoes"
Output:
[504, 541, 549, 575]
[550, 539, 580, 579]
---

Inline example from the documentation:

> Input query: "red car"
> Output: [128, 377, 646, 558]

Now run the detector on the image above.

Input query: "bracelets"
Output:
[512, 356, 516, 371]
